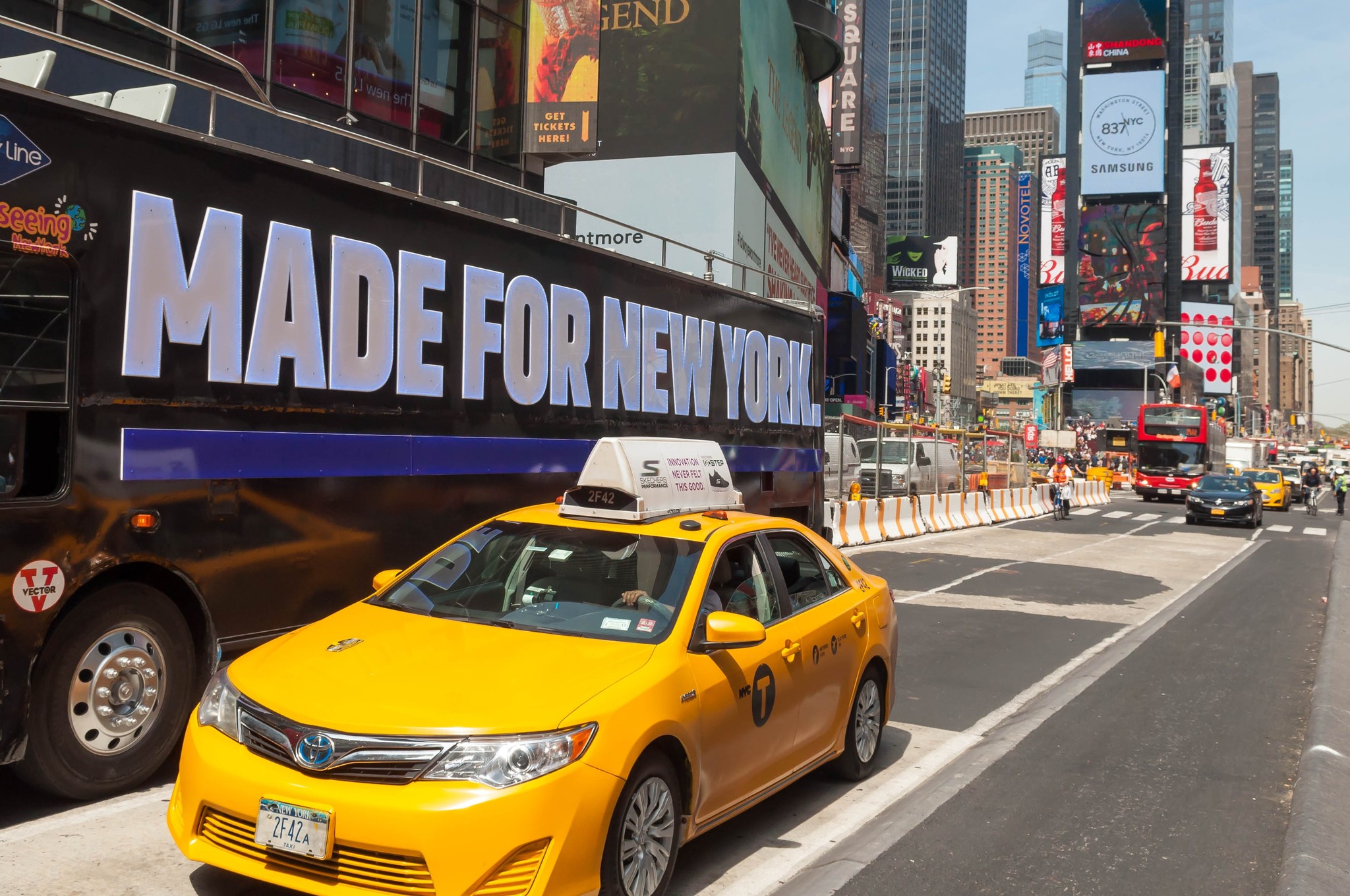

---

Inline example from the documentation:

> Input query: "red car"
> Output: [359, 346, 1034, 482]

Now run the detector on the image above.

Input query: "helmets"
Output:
[1057, 457, 1065, 463]
[1335, 468, 1344, 475]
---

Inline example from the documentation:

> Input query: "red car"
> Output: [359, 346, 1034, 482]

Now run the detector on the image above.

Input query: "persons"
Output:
[888, 236, 948, 282]
[901, 412, 1350, 516]
[1045, 457, 1073, 516]
[1303, 467, 1321, 498]
[621, 552, 723, 639]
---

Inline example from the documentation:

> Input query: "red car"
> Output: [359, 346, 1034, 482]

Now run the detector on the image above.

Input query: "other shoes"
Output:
[1065, 510, 1069, 516]
[1304, 499, 1307, 504]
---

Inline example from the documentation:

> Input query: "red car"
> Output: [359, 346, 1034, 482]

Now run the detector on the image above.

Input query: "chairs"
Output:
[712, 553, 748, 616]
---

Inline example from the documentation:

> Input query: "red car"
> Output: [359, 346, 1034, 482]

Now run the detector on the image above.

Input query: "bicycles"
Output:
[1303, 484, 1321, 516]
[1047, 475, 1070, 521]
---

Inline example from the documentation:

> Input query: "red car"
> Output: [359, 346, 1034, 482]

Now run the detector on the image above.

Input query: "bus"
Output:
[1134, 402, 1228, 502]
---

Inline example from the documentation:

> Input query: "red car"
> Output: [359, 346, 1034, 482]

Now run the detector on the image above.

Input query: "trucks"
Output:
[0, 1, 831, 807]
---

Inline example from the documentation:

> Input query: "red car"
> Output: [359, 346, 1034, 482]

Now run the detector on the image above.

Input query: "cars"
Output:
[169, 431, 902, 896]
[1237, 468, 1292, 512]
[1185, 475, 1263, 529]
[1225, 437, 1350, 512]
[1263, 465, 1305, 503]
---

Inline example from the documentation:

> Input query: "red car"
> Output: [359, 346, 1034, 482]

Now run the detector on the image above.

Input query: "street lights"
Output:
[890, 287, 991, 439]
[1114, 360, 1179, 404]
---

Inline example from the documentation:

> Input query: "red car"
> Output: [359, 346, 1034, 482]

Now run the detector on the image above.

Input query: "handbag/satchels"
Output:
[1059, 485, 1071, 500]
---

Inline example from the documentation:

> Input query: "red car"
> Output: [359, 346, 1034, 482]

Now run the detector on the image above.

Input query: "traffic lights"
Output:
[942, 373, 952, 394]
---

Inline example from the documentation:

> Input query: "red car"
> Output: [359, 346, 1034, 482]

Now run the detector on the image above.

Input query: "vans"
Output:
[855, 435, 962, 499]
[824, 431, 862, 502]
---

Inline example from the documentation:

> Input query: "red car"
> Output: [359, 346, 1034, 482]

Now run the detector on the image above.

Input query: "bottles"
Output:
[1051, 168, 1066, 256]
[1194, 159, 1218, 251]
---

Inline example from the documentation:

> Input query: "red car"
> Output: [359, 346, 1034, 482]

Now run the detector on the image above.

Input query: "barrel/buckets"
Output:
[1086, 467, 1113, 497]
